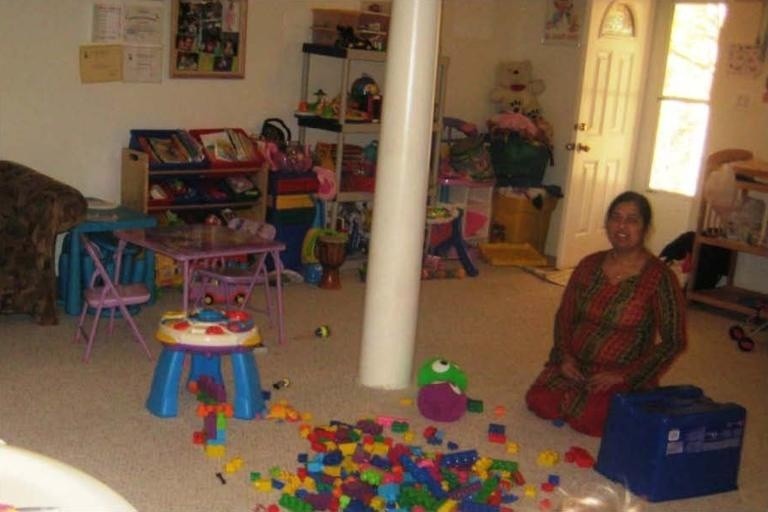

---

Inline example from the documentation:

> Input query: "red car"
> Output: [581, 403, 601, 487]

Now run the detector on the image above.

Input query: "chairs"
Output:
[71, 232, 156, 367]
[188, 216, 278, 332]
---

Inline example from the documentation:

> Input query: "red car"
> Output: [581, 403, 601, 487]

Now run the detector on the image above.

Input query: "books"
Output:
[140, 129, 256, 164]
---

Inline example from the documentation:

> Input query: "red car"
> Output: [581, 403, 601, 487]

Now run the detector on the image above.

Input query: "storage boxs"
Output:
[491, 188, 559, 261]
[592, 384, 747, 504]
[307, 26, 387, 51]
[309, 6, 390, 33]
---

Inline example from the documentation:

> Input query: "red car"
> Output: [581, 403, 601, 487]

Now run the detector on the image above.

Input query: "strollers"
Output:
[727, 296, 768, 353]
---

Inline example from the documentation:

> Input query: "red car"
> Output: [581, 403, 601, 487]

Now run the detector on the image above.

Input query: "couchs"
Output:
[0, 158, 88, 330]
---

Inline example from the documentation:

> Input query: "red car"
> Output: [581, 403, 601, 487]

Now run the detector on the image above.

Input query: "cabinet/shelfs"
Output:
[116, 143, 271, 244]
[675, 141, 767, 328]
[292, 39, 454, 253]
[439, 169, 498, 249]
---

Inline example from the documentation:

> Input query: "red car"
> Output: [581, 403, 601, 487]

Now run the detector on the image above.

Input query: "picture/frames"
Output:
[167, 0, 249, 82]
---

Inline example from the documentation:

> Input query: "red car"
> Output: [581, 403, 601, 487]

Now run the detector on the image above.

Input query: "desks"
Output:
[54, 200, 159, 319]
[103, 223, 289, 369]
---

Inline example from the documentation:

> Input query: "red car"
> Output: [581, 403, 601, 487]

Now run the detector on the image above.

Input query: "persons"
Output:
[546, 0, 577, 32]
[524, 190, 689, 437]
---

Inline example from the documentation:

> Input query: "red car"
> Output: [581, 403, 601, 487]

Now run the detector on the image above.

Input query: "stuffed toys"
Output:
[489, 60, 545, 118]
[416, 353, 468, 422]
